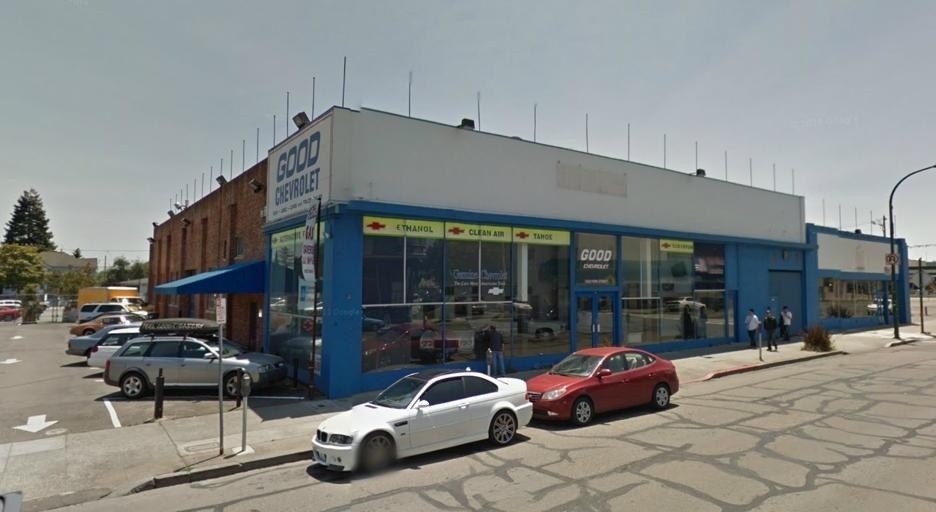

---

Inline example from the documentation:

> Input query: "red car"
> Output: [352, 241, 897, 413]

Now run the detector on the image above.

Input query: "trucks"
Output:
[76, 285, 161, 321]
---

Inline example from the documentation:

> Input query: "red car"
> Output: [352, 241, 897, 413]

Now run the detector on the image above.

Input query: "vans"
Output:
[87, 328, 143, 369]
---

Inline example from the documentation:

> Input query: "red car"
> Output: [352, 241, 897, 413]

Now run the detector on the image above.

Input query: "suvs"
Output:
[77, 302, 161, 324]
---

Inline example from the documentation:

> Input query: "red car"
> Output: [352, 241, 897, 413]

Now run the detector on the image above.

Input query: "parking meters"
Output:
[238, 372, 253, 452]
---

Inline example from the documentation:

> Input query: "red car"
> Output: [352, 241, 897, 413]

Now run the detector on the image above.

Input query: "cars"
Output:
[526, 346, 679, 427]
[0, 299, 50, 322]
[310, 369, 535, 477]
[65, 321, 145, 356]
[68, 312, 147, 337]
[464, 311, 565, 343]
[287, 311, 473, 371]
[104, 317, 288, 399]
[866, 297, 893, 317]
[665, 295, 708, 313]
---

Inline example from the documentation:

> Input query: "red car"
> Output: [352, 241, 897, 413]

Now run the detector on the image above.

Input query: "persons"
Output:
[680, 304, 708, 340]
[744, 305, 793, 352]
[423, 315, 434, 332]
[484, 325, 506, 378]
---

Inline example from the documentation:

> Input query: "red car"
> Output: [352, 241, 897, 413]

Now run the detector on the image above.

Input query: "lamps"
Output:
[457, 118, 475, 129]
[216, 176, 226, 186]
[180, 218, 189, 226]
[292, 112, 309, 128]
[692, 170, 705, 179]
[247, 179, 262, 194]
